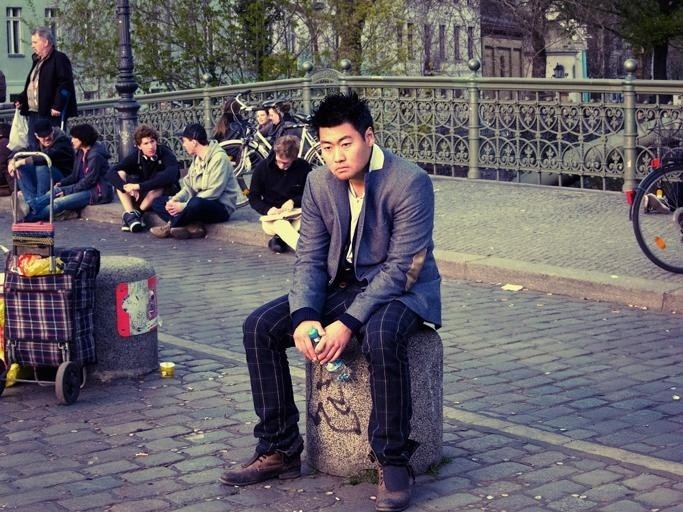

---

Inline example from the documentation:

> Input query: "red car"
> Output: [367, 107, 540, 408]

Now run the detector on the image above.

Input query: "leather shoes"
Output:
[219, 451, 301, 486]
[367, 440, 420, 511]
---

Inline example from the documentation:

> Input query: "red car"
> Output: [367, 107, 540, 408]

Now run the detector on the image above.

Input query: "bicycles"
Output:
[624, 157, 682, 276]
[220, 90, 325, 210]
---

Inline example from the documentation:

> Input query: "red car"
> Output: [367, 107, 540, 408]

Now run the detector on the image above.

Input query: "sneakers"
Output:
[12, 190, 28, 221]
[122, 211, 205, 240]
[268, 234, 282, 251]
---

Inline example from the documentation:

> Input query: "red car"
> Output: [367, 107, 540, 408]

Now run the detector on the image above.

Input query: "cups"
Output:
[158, 361, 176, 379]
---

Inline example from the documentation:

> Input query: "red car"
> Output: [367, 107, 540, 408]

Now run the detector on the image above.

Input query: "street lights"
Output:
[553, 62, 565, 103]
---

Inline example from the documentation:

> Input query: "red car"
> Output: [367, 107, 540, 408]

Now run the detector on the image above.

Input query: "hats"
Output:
[174, 124, 207, 140]
[35, 118, 53, 137]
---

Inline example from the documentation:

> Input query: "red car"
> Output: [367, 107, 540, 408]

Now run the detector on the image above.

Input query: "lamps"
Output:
[551, 62, 565, 79]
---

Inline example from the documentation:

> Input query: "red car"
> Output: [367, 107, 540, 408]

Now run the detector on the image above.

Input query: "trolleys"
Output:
[0, 150, 99, 405]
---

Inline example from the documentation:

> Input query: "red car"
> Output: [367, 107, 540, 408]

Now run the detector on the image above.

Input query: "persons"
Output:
[216, 84, 443, 512]
[104, 124, 183, 233]
[14, 28, 80, 151]
[150, 122, 237, 240]
[211, 108, 299, 159]
[247, 137, 314, 252]
[8, 118, 114, 224]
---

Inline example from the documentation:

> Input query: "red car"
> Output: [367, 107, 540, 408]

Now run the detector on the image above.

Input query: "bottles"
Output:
[306, 327, 354, 382]
